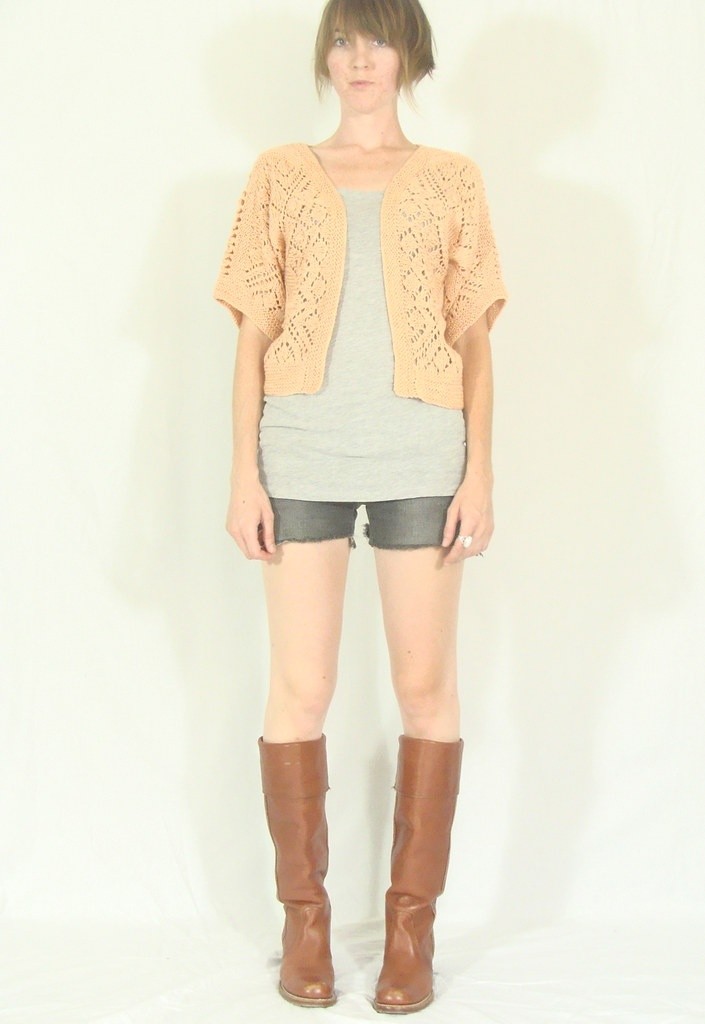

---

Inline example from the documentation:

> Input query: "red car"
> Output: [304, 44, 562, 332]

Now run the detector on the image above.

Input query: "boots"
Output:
[374, 734, 464, 1015]
[258, 734, 337, 1008]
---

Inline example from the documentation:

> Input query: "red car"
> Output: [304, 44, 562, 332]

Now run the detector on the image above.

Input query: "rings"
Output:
[457, 533, 473, 550]
[476, 550, 489, 560]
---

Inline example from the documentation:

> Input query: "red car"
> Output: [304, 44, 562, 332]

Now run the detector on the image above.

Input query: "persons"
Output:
[213, 0, 509, 1012]
